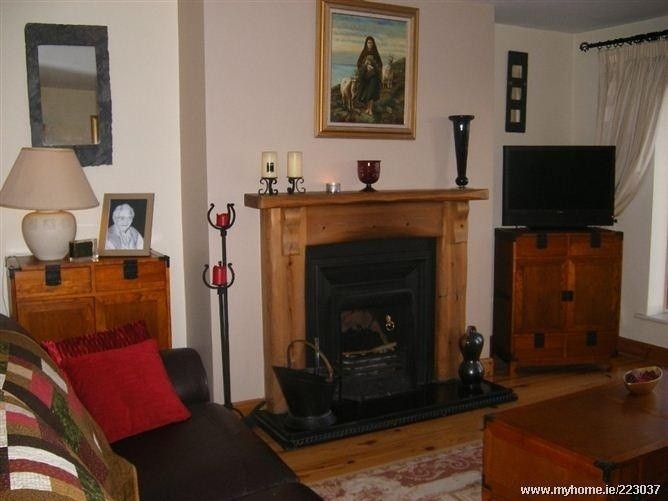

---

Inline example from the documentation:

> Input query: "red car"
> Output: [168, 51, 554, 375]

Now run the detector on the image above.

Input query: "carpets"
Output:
[301, 439, 483, 501]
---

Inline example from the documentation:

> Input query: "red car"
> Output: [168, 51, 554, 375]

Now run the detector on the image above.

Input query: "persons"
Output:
[358, 37, 383, 116]
[106, 205, 145, 249]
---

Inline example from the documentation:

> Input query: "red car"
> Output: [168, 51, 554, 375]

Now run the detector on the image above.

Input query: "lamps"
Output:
[1, 147, 99, 261]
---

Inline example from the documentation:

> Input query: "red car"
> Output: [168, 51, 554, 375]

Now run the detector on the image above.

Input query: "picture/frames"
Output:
[315, 0, 420, 142]
[96, 194, 153, 259]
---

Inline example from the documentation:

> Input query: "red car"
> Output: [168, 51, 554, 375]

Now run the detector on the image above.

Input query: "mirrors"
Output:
[25, 22, 112, 167]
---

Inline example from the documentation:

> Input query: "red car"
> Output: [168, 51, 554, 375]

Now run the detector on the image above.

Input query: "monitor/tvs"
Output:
[501, 145, 617, 231]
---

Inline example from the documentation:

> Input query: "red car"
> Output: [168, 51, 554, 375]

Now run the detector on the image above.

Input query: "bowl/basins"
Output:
[623, 366, 663, 393]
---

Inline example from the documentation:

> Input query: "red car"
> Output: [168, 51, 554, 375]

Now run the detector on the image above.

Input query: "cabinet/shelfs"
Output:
[4, 250, 173, 355]
[494, 227, 624, 375]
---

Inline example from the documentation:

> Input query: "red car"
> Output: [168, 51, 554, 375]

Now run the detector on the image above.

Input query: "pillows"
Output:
[40, 321, 191, 444]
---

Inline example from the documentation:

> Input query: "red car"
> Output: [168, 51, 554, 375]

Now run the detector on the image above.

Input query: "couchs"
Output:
[0, 313, 324, 501]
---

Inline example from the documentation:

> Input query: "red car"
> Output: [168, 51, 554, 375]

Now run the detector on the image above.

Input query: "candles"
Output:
[286, 151, 304, 177]
[260, 149, 280, 179]
[216, 213, 226, 229]
[212, 265, 226, 286]
